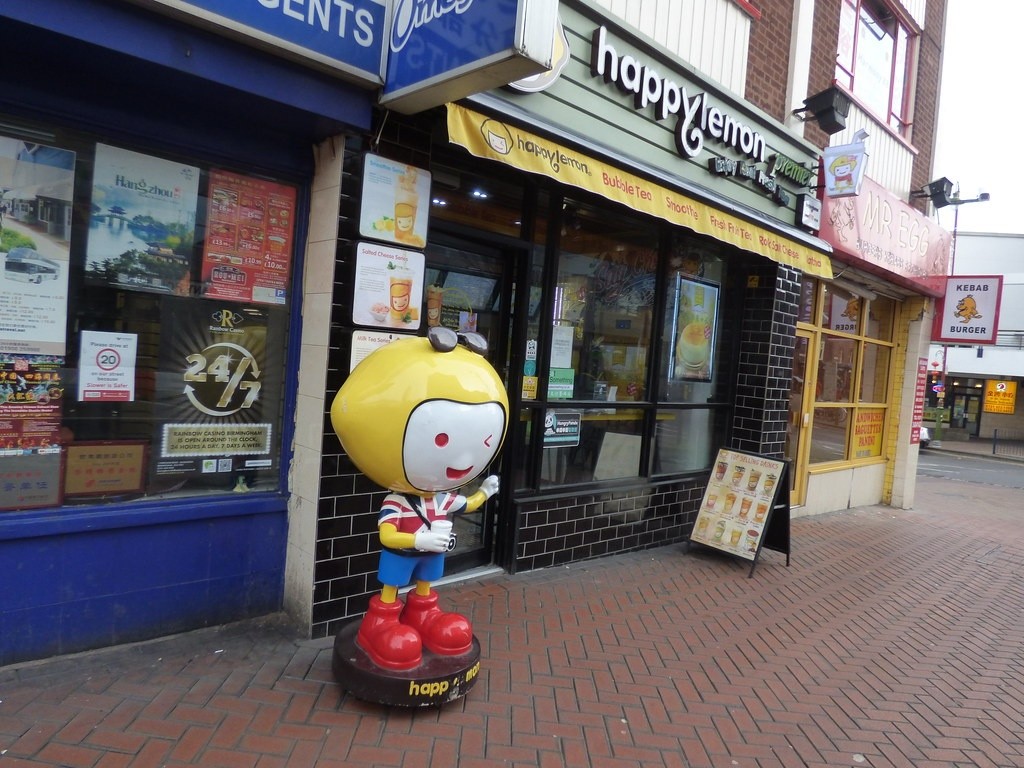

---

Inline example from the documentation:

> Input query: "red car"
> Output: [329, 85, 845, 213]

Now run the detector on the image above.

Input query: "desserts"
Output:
[679, 323, 709, 365]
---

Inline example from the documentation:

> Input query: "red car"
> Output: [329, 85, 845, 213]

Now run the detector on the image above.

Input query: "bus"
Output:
[4, 246, 60, 284]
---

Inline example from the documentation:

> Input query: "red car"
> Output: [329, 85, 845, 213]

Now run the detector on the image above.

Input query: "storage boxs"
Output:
[599, 344, 647, 398]
[598, 309, 652, 346]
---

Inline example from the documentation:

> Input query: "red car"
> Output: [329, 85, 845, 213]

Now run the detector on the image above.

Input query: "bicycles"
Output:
[814, 400, 847, 423]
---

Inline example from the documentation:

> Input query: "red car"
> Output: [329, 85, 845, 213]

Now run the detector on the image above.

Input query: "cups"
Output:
[394, 182, 419, 240]
[385, 266, 416, 322]
[459, 311, 477, 333]
[697, 461, 777, 551]
[427, 286, 442, 327]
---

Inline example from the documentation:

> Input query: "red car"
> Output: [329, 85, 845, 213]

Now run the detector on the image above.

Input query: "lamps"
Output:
[793, 86, 853, 136]
[910, 177, 954, 210]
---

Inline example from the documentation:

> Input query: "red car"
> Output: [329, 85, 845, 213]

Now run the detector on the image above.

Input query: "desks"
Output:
[521, 408, 677, 485]
